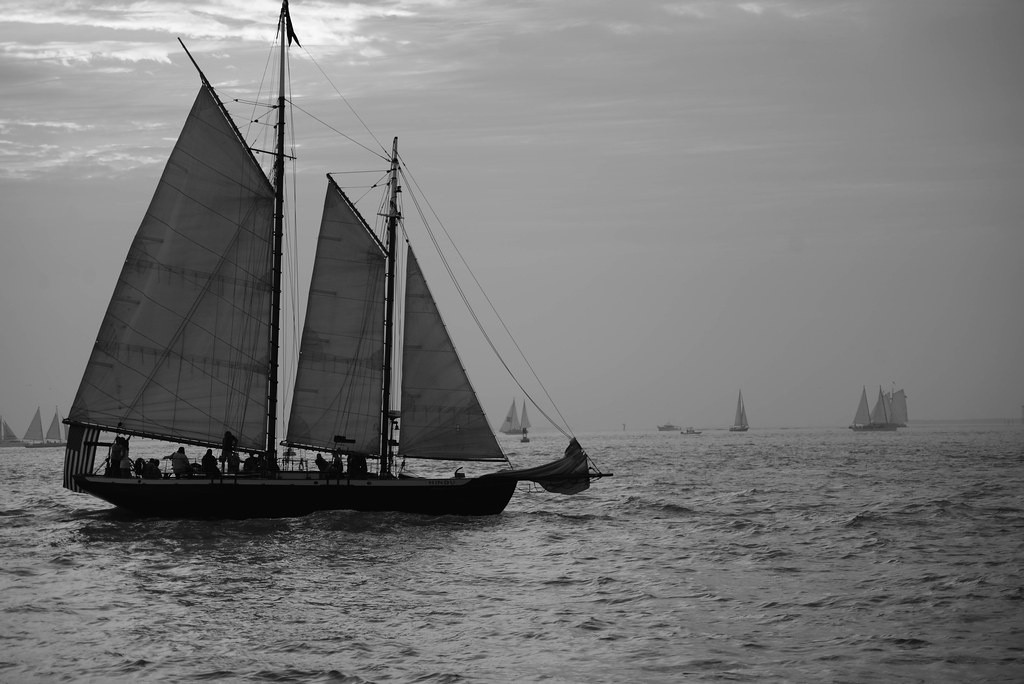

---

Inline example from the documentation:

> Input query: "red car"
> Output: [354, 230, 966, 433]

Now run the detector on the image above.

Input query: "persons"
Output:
[108, 430, 581, 478]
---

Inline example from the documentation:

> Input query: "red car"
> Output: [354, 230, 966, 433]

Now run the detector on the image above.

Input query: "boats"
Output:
[681, 426, 701, 435]
[656, 424, 681, 431]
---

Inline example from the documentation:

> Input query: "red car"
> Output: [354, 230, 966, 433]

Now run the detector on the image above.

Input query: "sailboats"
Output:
[847, 382, 912, 432]
[498, 394, 531, 436]
[0, 402, 73, 449]
[729, 387, 750, 432]
[54, 0, 624, 521]
